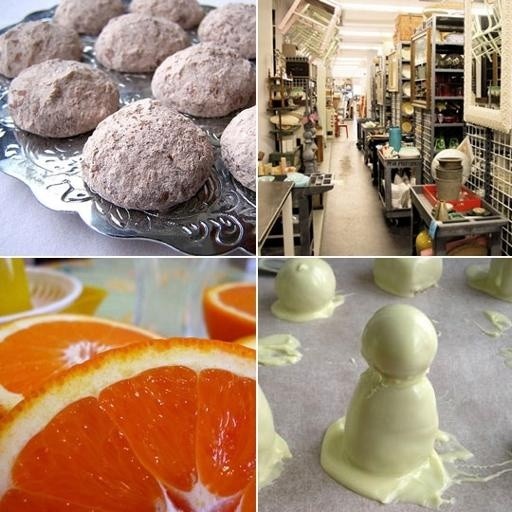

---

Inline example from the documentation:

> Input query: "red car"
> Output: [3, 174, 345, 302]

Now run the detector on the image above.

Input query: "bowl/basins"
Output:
[424, 10, 449, 18]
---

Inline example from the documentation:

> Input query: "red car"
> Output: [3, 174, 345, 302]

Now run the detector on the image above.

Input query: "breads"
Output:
[0, 0, 256, 208]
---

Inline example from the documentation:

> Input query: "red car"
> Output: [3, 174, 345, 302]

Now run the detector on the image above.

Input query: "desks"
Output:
[315, 128, 323, 162]
[258, 181, 295, 256]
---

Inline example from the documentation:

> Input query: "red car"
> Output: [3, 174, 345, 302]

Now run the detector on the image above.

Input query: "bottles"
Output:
[389, 127, 401, 152]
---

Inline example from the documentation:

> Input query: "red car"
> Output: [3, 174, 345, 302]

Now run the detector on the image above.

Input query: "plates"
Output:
[402, 46, 415, 133]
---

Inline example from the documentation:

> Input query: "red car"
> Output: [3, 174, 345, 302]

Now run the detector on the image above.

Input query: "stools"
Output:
[278, 0, 343, 56]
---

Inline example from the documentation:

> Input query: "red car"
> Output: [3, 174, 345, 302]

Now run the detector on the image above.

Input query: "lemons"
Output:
[1, 281, 256, 512]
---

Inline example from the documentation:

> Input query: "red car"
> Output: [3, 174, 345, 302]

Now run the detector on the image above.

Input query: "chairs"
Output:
[334, 114, 348, 138]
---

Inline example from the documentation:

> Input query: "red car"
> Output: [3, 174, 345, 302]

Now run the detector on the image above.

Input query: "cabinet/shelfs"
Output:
[433, 17, 465, 153]
[384, 56, 391, 127]
[267, 105, 305, 174]
[368, 132, 406, 185]
[410, 184, 508, 256]
[377, 146, 423, 226]
[400, 42, 415, 145]
